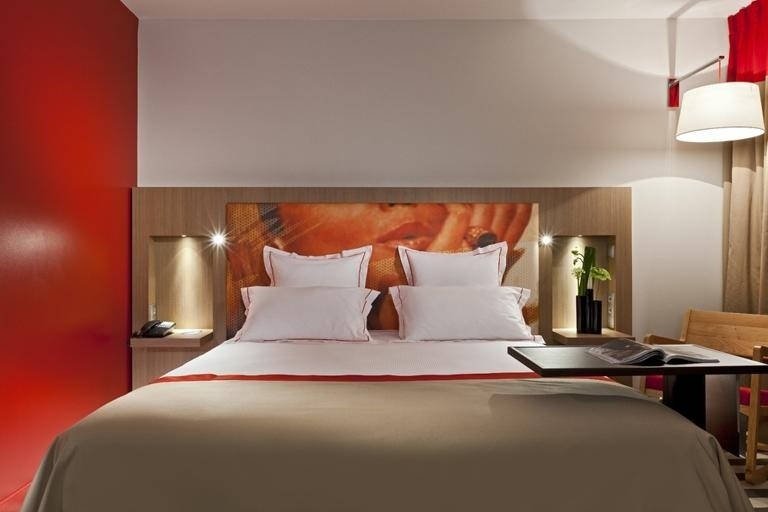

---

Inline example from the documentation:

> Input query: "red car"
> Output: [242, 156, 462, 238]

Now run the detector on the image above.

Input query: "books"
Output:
[584, 337, 721, 365]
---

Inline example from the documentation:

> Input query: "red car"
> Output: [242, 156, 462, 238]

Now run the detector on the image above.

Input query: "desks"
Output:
[507, 341, 768, 480]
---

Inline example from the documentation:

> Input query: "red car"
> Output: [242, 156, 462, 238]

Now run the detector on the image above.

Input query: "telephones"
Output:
[133, 319, 176, 338]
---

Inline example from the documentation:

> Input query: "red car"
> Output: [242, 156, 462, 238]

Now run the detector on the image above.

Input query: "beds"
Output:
[22, 202, 754, 512]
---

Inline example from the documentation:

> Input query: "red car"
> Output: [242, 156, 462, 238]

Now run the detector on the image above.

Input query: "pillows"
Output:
[262, 245, 372, 287]
[398, 241, 508, 286]
[389, 284, 535, 342]
[234, 287, 380, 342]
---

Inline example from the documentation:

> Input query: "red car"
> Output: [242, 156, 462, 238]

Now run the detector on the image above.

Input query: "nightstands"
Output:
[548, 325, 638, 350]
[129, 324, 214, 390]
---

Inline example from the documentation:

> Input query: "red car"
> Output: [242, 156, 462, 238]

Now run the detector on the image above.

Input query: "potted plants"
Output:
[572, 247, 612, 334]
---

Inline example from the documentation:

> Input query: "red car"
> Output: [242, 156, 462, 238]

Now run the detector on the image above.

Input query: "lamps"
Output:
[669, 56, 764, 143]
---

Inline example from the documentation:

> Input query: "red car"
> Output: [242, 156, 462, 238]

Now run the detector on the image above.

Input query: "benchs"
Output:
[640, 309, 768, 482]
